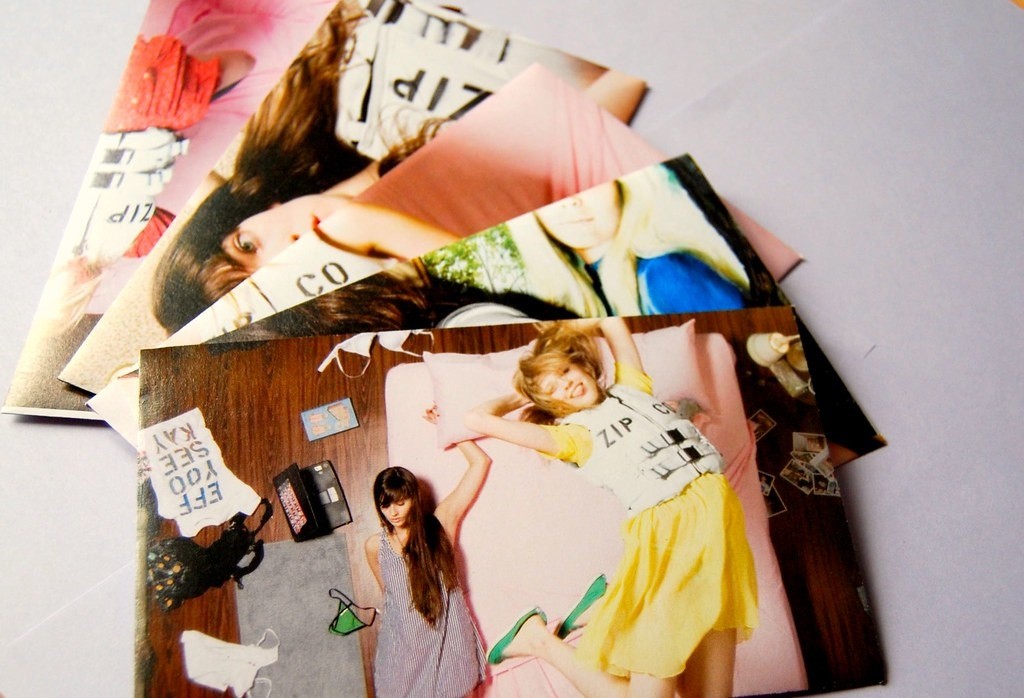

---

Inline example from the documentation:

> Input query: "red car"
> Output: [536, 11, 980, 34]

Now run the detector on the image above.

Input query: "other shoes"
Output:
[552, 572, 608, 640]
[485, 606, 548, 665]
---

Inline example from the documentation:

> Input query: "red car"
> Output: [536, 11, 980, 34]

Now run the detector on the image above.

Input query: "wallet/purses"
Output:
[272, 460, 354, 543]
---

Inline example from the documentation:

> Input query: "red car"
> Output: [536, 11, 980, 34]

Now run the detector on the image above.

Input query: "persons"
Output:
[467, 317, 763, 698]
[149, 0, 451, 334]
[362, 399, 496, 698]
[507, 177, 748, 316]
